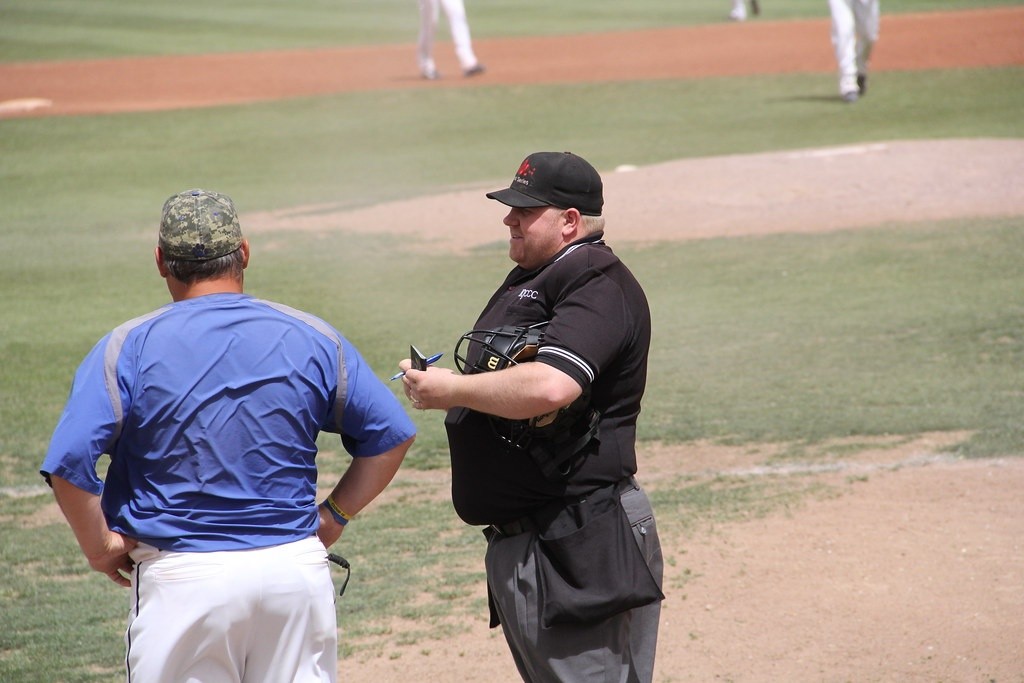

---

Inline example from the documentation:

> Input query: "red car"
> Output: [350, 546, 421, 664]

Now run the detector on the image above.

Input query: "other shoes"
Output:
[843, 93, 857, 102]
[854, 73, 867, 96]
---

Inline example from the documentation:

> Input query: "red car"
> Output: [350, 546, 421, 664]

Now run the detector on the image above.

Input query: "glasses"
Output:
[329, 553, 350, 597]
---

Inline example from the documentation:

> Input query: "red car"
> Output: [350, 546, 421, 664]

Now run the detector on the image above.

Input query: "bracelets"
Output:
[323, 494, 351, 526]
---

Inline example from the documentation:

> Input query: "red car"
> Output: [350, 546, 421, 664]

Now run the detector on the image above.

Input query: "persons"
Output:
[416, 0, 486, 79]
[40, 188, 416, 683]
[398, 151, 664, 683]
[827, 0, 881, 103]
[728, 0, 762, 22]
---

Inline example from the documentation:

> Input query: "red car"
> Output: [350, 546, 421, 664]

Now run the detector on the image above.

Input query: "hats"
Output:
[485, 152, 604, 217]
[160, 189, 242, 261]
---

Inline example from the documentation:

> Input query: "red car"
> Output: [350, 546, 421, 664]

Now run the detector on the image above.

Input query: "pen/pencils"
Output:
[389, 352, 443, 382]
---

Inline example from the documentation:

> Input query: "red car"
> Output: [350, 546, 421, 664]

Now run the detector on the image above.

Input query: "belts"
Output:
[490, 471, 638, 538]
